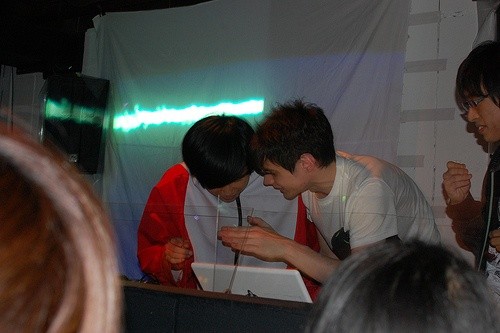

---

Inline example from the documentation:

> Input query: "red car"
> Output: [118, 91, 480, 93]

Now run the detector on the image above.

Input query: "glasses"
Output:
[461, 93, 489, 112]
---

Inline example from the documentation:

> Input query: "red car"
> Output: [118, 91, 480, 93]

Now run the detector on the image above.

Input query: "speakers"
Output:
[41, 75, 110, 174]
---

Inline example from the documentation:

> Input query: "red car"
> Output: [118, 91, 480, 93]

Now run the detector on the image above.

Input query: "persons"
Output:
[137, 114, 322, 304]
[442, 40, 500, 273]
[302, 236, 498, 333]
[218, 97, 441, 288]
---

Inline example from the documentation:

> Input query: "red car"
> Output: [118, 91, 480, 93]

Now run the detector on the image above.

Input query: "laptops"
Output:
[190, 262, 313, 304]
